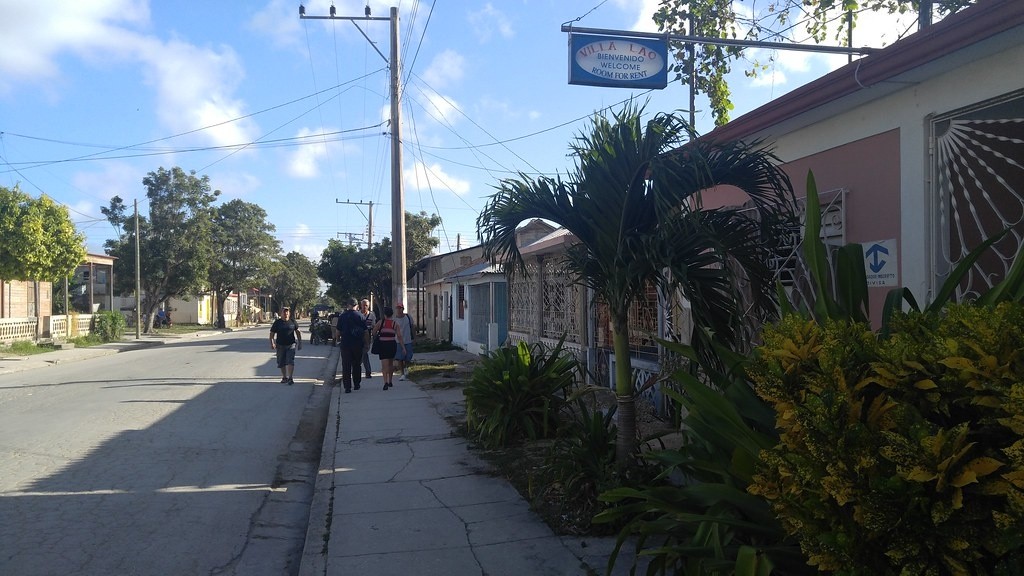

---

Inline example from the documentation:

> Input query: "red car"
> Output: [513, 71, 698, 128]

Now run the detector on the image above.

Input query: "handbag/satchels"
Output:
[372, 319, 384, 354]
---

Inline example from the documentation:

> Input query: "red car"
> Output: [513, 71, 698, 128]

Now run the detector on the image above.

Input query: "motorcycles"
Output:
[310, 313, 343, 347]
[124, 308, 173, 329]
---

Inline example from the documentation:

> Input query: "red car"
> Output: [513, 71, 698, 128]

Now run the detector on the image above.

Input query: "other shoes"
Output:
[289, 379, 293, 385]
[399, 375, 405, 381]
[365, 374, 371, 378]
[345, 389, 351, 393]
[404, 368, 409, 376]
[354, 385, 360, 390]
[388, 383, 392, 387]
[383, 383, 388, 390]
[281, 378, 287, 383]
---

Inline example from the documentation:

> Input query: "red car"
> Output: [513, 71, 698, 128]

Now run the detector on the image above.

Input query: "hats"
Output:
[281, 307, 288, 312]
[347, 297, 358, 305]
[395, 303, 403, 308]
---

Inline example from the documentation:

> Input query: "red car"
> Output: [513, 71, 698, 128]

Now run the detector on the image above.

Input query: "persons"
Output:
[330, 313, 338, 323]
[358, 299, 377, 378]
[274, 312, 278, 321]
[392, 302, 416, 381]
[158, 308, 171, 328]
[335, 296, 371, 392]
[372, 307, 406, 391]
[310, 312, 319, 341]
[270, 307, 301, 384]
